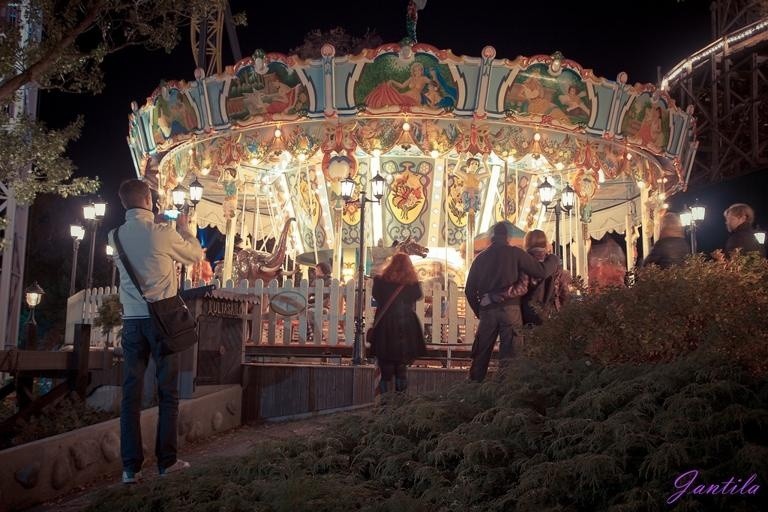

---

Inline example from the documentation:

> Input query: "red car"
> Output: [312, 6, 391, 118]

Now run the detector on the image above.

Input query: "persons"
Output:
[106, 180, 205, 483]
[464, 222, 569, 383]
[363, 252, 429, 408]
[720, 203, 763, 255]
[123, 39, 700, 267]
[423, 262, 450, 343]
[308, 262, 332, 340]
[641, 213, 698, 265]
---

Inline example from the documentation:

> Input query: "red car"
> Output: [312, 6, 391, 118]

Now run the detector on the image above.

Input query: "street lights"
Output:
[24, 280, 46, 349]
[104, 244, 120, 293]
[69, 217, 86, 296]
[537, 176, 576, 256]
[340, 170, 387, 365]
[754, 223, 768, 253]
[171, 177, 204, 291]
[80, 196, 107, 288]
[679, 198, 707, 263]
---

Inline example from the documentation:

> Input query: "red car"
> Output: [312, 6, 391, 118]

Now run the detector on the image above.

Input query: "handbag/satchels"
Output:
[364, 326, 376, 358]
[146, 292, 199, 354]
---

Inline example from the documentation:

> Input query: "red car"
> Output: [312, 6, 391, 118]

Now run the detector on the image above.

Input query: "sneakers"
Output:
[122, 467, 144, 483]
[159, 458, 190, 475]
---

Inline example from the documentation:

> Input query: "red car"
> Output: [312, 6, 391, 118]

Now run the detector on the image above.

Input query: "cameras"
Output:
[165, 210, 178, 220]
